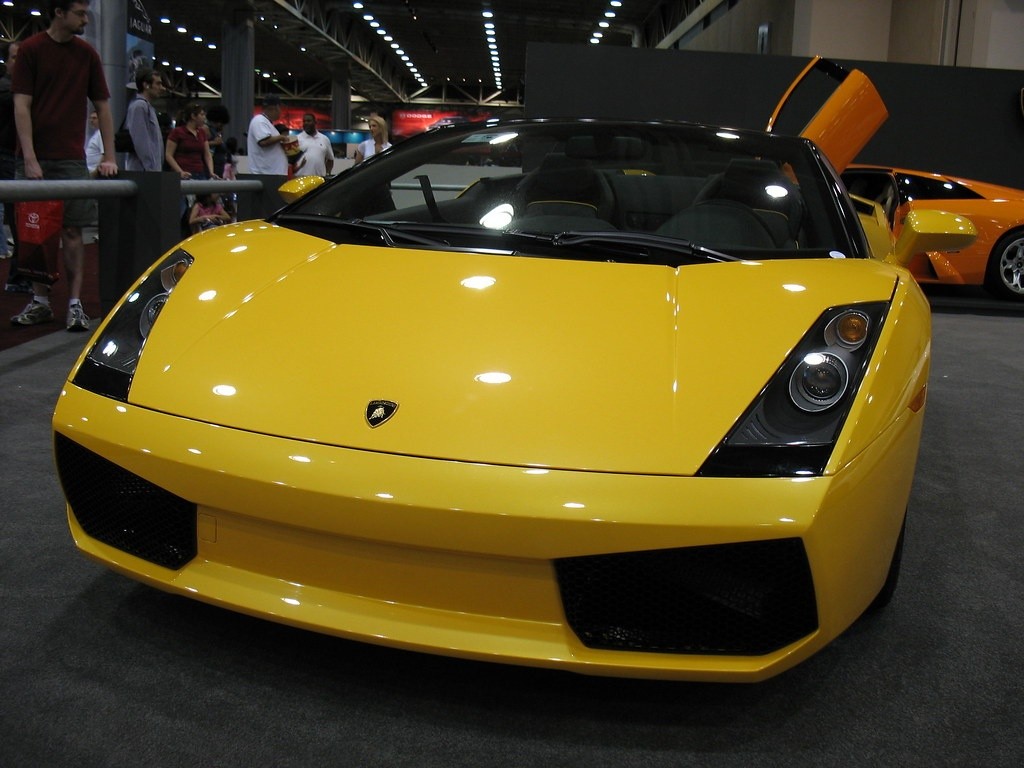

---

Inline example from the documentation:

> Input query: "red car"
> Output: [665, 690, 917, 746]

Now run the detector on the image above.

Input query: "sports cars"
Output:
[757, 55, 1024, 306]
[52, 119, 978, 683]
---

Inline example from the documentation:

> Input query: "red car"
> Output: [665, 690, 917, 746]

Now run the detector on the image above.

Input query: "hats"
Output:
[158, 113, 171, 125]
[205, 105, 232, 125]
[262, 95, 281, 108]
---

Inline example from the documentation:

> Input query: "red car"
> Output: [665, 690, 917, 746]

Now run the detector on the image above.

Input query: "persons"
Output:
[275, 123, 306, 181]
[86, 112, 105, 178]
[247, 96, 290, 175]
[0, 42, 52, 294]
[355, 117, 393, 164]
[293, 114, 334, 176]
[11, 0, 117, 331]
[125, 69, 164, 171]
[166, 102, 231, 235]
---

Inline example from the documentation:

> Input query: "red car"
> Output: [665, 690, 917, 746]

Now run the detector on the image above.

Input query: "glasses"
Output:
[67, 7, 91, 18]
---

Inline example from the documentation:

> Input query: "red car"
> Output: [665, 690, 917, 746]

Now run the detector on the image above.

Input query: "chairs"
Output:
[701, 157, 805, 237]
[503, 135, 617, 222]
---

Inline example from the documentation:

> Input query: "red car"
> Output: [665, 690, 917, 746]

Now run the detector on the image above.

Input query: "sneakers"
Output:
[66, 306, 91, 331]
[10, 302, 55, 326]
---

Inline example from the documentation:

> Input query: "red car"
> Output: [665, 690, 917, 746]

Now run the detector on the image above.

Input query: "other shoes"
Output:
[6, 275, 51, 294]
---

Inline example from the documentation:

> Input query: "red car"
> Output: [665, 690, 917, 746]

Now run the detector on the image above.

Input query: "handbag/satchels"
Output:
[115, 128, 132, 153]
[15, 201, 64, 245]
